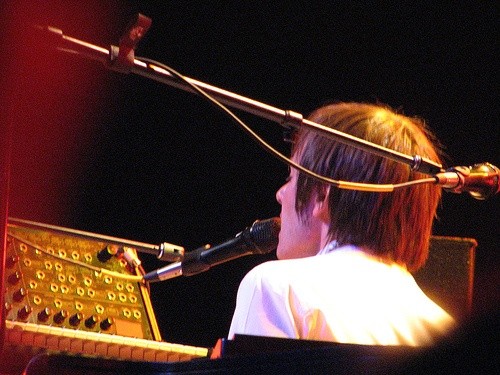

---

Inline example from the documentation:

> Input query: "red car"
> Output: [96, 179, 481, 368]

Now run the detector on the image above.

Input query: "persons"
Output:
[227, 102, 460, 350]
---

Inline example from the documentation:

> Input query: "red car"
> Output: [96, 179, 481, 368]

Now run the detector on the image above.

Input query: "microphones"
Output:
[144, 216, 281, 282]
[433, 162, 500, 201]
